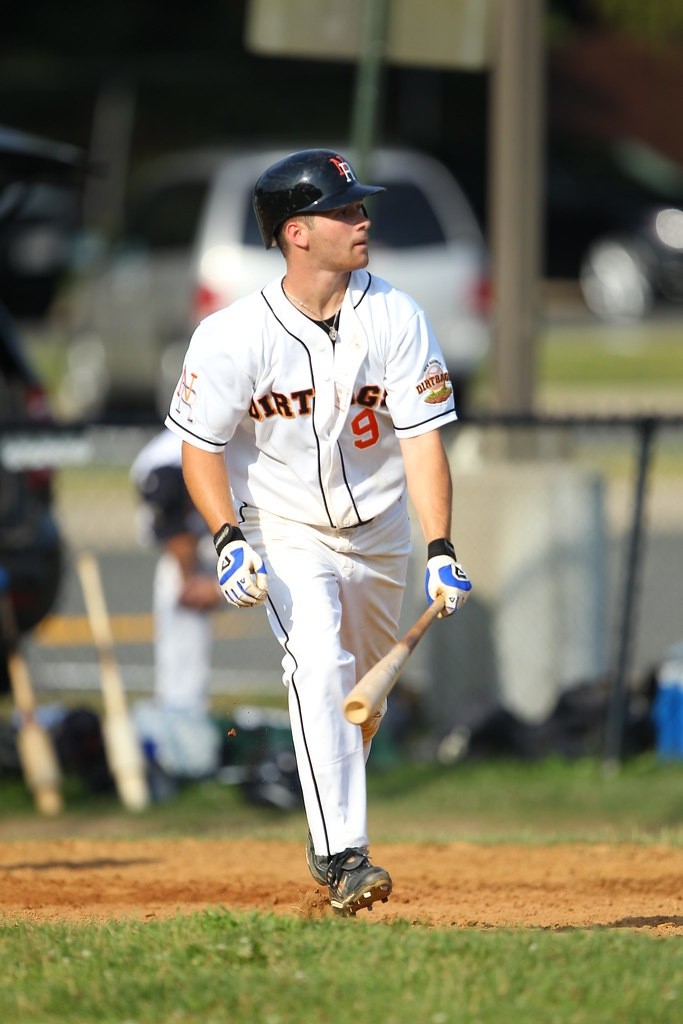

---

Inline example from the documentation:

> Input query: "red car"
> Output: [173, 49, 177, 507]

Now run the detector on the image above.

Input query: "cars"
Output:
[191, 146, 491, 415]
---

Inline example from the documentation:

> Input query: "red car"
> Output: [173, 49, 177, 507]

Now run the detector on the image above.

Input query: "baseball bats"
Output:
[76, 554, 152, 812]
[344, 591, 444, 723]
[1, 567, 64, 814]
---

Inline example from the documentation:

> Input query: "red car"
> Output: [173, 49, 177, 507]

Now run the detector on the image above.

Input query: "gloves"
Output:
[424, 537, 472, 619]
[213, 523, 268, 608]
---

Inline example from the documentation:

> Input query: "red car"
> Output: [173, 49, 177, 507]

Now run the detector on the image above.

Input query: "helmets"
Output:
[255, 149, 388, 249]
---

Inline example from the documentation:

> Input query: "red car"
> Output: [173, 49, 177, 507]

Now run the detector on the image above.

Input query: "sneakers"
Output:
[326, 848, 392, 918]
[305, 829, 331, 887]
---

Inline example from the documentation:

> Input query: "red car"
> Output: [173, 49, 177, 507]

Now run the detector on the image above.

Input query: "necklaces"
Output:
[280, 281, 342, 341]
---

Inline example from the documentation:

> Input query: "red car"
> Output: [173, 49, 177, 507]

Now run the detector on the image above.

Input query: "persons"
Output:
[125, 421, 286, 809]
[173, 143, 474, 918]
